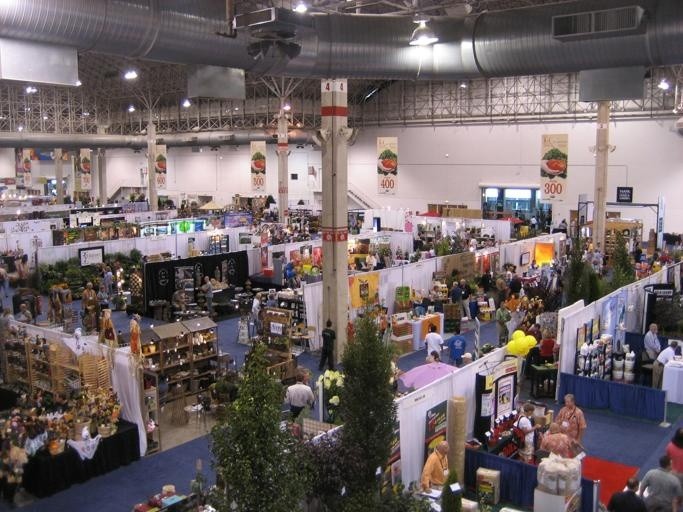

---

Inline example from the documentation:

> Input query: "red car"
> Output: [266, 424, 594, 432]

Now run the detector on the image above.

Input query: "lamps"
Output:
[76, 80, 82, 87]
[183, 97, 192, 108]
[124, 62, 141, 82]
[657, 75, 673, 92]
[128, 103, 136, 114]
[283, 99, 293, 113]
[409, 13, 439, 47]
[26, 85, 39, 95]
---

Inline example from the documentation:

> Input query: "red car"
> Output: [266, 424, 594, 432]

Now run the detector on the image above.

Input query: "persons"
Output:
[251, 291, 262, 332]
[318, 320, 336, 371]
[459, 227, 493, 252]
[526, 208, 552, 229]
[582, 237, 607, 279]
[607, 427, 680, 509]
[517, 394, 587, 459]
[82, 262, 114, 317]
[15, 303, 32, 323]
[632, 239, 675, 280]
[645, 325, 677, 389]
[254, 206, 312, 246]
[415, 278, 472, 320]
[366, 250, 381, 271]
[422, 441, 449, 494]
[0, 184, 683, 511]
[350, 214, 358, 229]
[17, 254, 31, 287]
[284, 372, 315, 420]
[269, 294, 278, 307]
[424, 325, 467, 370]
[172, 289, 188, 311]
[202, 275, 230, 310]
[480, 259, 555, 398]
[559, 219, 578, 237]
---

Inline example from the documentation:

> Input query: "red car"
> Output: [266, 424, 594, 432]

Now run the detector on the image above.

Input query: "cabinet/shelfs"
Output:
[0, 195, 682, 512]
